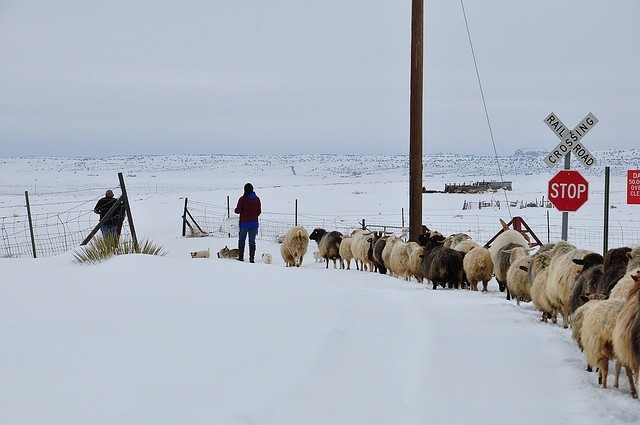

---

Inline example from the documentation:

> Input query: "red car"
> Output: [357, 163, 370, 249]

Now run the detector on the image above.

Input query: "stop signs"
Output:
[549, 169, 590, 213]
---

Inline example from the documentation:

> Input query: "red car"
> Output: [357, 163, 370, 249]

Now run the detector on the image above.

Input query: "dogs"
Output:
[189, 247, 212, 258]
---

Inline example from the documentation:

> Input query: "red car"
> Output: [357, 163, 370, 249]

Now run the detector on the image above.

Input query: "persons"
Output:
[116, 196, 125, 237]
[235, 183, 261, 263]
[94, 190, 119, 247]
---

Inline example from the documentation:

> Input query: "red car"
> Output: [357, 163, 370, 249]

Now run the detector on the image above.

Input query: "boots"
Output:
[250, 246, 255, 263]
[236, 247, 244, 261]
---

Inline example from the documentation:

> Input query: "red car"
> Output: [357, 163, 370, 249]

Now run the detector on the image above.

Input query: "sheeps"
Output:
[390, 232, 423, 287]
[463, 244, 492, 292]
[547, 239, 592, 328]
[489, 217, 530, 300]
[610, 244, 640, 301]
[313, 250, 324, 264]
[568, 292, 639, 402]
[261, 252, 273, 264]
[280, 241, 303, 268]
[568, 252, 605, 312]
[284, 225, 309, 267]
[612, 269, 640, 390]
[604, 247, 633, 299]
[501, 246, 538, 306]
[339, 234, 360, 272]
[350, 228, 374, 272]
[436, 231, 481, 255]
[364, 230, 394, 275]
[219, 246, 240, 260]
[309, 227, 345, 270]
[419, 247, 462, 291]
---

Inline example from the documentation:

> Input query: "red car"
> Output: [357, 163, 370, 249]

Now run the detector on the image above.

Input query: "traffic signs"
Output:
[626, 169, 640, 205]
